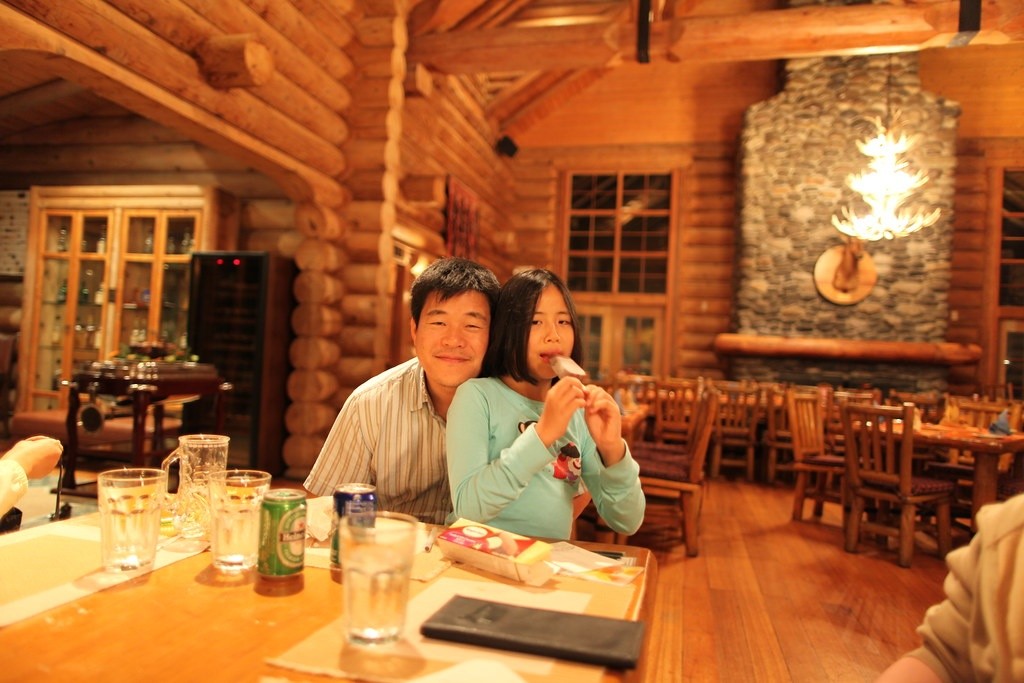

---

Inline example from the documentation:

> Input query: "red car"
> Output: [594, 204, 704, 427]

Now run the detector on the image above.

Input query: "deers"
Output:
[828, 227, 866, 292]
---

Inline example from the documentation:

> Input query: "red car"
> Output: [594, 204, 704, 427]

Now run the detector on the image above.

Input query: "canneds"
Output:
[329, 484, 377, 565]
[258, 489, 308, 578]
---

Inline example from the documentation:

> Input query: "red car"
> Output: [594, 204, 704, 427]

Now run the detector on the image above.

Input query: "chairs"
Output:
[584, 371, 1024, 568]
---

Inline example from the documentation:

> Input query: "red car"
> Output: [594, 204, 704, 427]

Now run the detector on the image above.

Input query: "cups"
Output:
[209, 470, 273, 571]
[98, 468, 165, 573]
[162, 435, 230, 540]
[339, 511, 419, 642]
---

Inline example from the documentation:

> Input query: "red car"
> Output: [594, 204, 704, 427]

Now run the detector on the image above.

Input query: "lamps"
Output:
[832, 51, 942, 242]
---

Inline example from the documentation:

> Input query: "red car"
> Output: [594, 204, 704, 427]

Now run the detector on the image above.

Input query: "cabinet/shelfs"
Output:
[181, 247, 294, 478]
[9, 184, 242, 454]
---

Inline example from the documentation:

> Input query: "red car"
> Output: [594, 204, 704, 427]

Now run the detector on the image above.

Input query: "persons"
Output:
[302, 256, 593, 523]
[0, 435, 64, 520]
[875, 492, 1024, 683]
[445, 266, 646, 540]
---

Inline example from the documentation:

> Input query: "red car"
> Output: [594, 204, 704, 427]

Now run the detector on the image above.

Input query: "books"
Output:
[420, 594, 645, 670]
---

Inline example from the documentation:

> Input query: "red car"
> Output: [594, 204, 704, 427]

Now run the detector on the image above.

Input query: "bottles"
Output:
[46, 225, 190, 393]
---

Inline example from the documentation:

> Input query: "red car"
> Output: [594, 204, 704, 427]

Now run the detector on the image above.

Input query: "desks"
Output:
[866, 417, 1023, 560]
[0, 486, 659, 683]
[58, 362, 231, 488]
